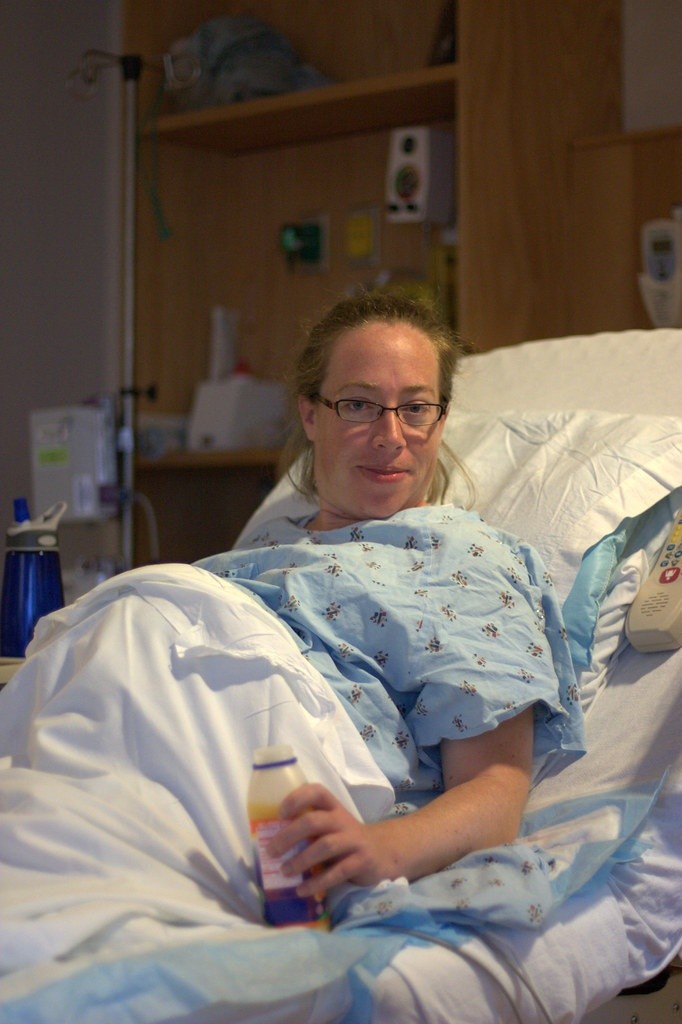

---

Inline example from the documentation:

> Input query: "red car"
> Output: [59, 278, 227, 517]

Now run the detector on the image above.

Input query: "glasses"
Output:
[310, 392, 447, 426]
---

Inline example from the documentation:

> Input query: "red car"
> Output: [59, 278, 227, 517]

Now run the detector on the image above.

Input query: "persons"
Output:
[188, 274, 590, 901]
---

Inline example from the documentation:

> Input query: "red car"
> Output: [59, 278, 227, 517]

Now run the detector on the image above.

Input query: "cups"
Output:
[1, 497, 68, 658]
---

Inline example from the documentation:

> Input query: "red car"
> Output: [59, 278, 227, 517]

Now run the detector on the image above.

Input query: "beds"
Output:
[0, 325, 680, 1024]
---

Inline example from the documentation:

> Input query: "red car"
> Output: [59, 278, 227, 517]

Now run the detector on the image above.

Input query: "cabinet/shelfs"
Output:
[112, 2, 625, 468]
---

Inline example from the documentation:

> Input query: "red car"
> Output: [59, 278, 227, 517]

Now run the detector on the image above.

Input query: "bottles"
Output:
[247, 741, 333, 936]
